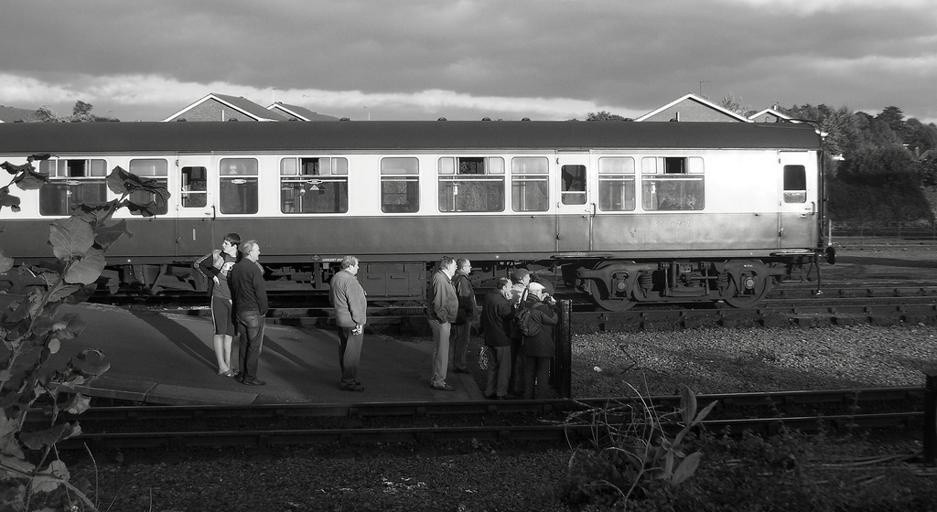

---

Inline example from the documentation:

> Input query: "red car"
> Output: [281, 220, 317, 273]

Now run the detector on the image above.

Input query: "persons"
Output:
[481, 267, 559, 400]
[230, 239, 269, 386]
[329, 255, 366, 391]
[426, 256, 459, 392]
[193, 232, 244, 377]
[451, 257, 479, 373]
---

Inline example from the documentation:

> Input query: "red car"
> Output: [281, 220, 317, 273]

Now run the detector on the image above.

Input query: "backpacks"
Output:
[513, 301, 546, 337]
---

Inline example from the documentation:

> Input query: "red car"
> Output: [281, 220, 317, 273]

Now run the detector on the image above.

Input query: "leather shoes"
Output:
[244, 378, 266, 386]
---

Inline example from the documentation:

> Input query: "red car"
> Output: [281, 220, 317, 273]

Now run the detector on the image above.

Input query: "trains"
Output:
[1, 117, 836, 325]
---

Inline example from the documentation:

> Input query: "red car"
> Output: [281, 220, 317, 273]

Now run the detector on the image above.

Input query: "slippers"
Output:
[216, 369, 239, 378]
